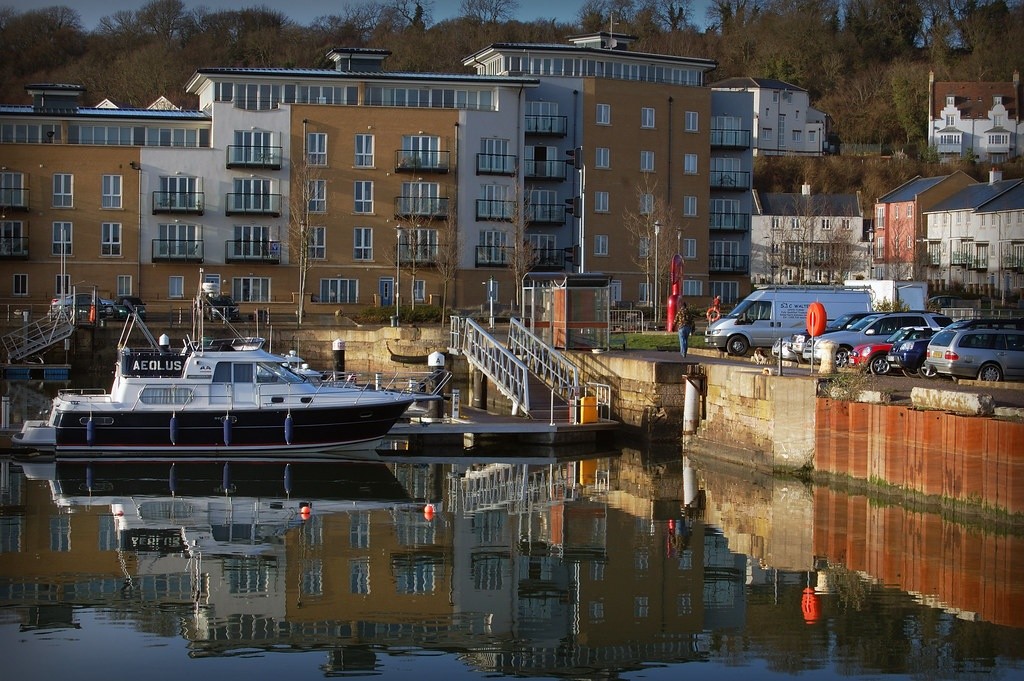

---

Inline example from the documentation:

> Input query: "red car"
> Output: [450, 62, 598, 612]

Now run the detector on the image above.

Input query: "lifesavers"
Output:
[704, 308, 722, 322]
[803, 301, 827, 337]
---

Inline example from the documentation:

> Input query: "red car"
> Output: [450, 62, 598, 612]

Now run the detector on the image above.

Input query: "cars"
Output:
[849, 327, 945, 375]
[928, 296, 963, 308]
[113, 294, 147, 322]
[195, 293, 240, 322]
[47, 293, 114, 320]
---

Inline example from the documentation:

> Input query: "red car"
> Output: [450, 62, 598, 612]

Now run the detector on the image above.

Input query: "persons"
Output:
[672, 301, 695, 358]
[670, 519, 690, 560]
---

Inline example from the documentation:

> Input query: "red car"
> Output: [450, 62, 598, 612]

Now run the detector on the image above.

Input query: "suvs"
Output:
[887, 318, 1024, 378]
[772, 310, 954, 368]
[926, 326, 1024, 382]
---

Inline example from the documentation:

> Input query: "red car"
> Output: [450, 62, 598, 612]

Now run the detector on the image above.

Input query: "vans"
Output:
[705, 283, 877, 356]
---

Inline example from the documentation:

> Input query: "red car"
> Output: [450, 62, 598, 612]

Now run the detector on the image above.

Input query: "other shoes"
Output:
[680, 353, 686, 357]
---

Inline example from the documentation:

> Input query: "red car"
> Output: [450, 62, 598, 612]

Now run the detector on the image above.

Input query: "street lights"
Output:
[394, 223, 407, 326]
[675, 226, 684, 255]
[866, 227, 876, 279]
[298, 220, 306, 308]
[654, 219, 663, 331]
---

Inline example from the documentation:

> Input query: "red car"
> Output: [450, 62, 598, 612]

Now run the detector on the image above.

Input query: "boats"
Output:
[10, 451, 427, 630]
[10, 270, 452, 455]
